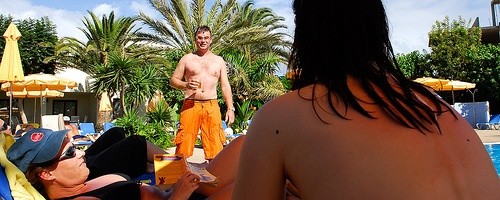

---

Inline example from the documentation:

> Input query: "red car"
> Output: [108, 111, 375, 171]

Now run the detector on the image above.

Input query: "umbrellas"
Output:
[0, 22, 78, 126]
[146, 88, 169, 113]
[410, 76, 476, 90]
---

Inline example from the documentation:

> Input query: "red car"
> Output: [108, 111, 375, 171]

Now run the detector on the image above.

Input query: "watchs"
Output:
[227, 107, 235, 111]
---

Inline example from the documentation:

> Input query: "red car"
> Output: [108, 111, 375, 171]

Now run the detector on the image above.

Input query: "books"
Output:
[155, 154, 220, 190]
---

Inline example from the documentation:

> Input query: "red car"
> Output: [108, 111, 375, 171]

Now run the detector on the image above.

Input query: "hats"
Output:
[6, 128, 71, 172]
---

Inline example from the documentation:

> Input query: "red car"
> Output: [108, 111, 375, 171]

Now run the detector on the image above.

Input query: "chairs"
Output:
[0, 115, 253, 200]
[476, 114, 500, 131]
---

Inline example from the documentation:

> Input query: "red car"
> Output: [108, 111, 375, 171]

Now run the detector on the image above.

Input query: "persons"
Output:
[6, 128, 301, 200]
[232, 0, 500, 199]
[171, 26, 235, 162]
[0, 118, 168, 184]
[63, 116, 88, 141]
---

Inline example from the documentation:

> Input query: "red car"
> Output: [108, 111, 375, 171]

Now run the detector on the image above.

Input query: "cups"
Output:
[191, 79, 202, 89]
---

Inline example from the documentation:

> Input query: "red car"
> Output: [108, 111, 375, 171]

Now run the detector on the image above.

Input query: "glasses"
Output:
[41, 143, 76, 167]
